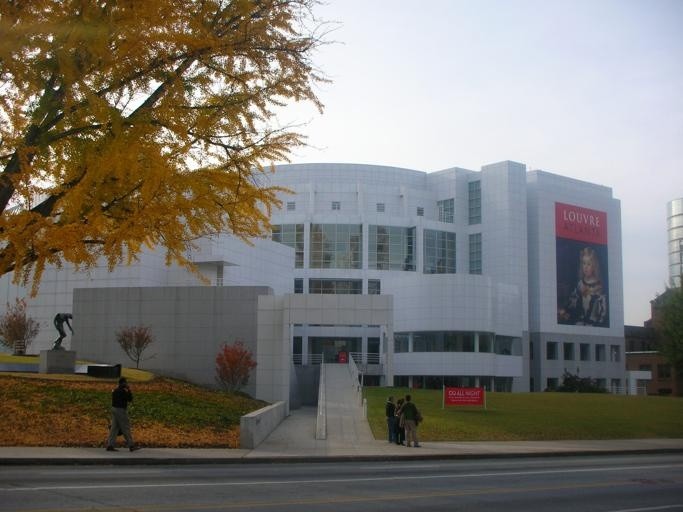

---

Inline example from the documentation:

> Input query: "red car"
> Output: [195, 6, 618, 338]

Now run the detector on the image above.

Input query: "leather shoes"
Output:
[107, 446, 120, 451]
[129, 446, 141, 452]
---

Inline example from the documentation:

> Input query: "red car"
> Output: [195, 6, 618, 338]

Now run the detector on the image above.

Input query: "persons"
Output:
[559, 246, 609, 327]
[396, 395, 422, 447]
[393, 399, 406, 446]
[385, 396, 396, 443]
[52, 313, 73, 350]
[107, 377, 140, 452]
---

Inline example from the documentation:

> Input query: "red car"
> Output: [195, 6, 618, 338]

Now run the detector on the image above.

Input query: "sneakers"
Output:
[389, 440, 421, 447]
